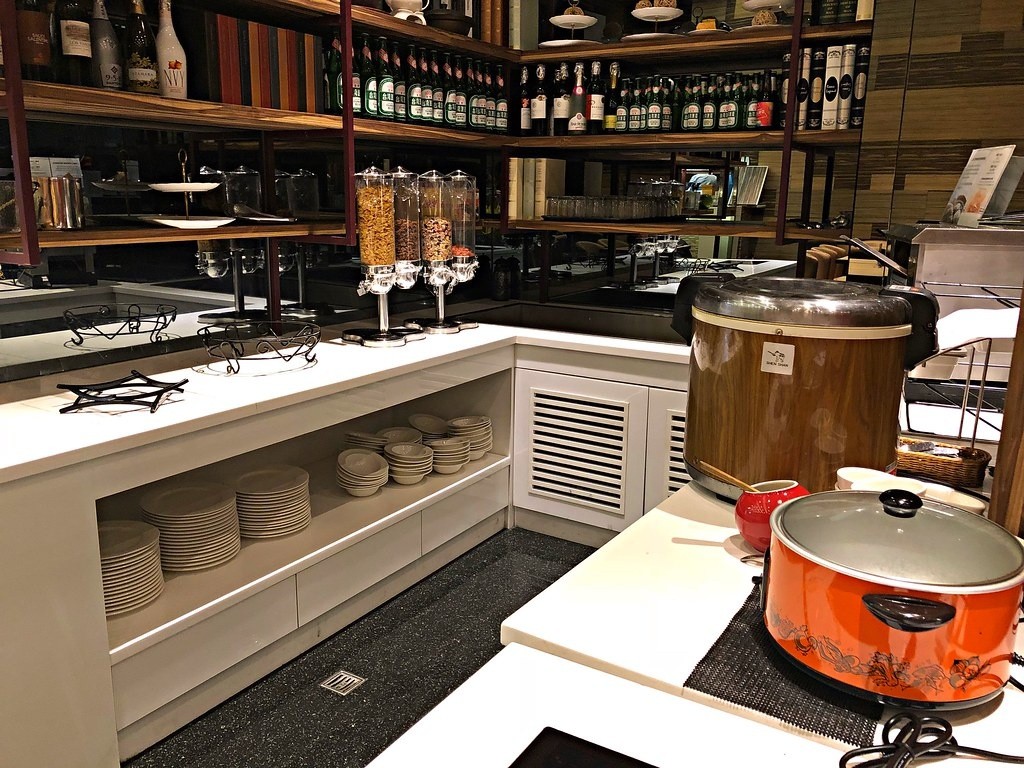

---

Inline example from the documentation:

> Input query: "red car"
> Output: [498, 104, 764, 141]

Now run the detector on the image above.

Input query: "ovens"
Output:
[837, 218, 1024, 319]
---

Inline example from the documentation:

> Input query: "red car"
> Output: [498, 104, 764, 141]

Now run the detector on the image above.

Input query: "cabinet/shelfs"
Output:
[0, 0, 876, 275]
[511, 345, 693, 549]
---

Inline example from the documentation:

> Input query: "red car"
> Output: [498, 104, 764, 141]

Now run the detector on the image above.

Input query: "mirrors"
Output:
[737, 166, 768, 205]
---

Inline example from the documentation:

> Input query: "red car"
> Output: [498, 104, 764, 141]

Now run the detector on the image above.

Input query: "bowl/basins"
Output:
[335, 413, 494, 497]
[733, 480, 811, 554]
[833, 467, 986, 516]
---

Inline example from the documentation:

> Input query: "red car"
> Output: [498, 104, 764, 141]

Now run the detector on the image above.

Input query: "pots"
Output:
[751, 489, 1024, 712]
[682, 276, 913, 506]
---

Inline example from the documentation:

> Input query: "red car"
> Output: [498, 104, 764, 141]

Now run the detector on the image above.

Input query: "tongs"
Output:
[223, 201, 298, 224]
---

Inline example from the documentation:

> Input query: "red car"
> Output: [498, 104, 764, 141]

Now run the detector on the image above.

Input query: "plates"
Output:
[152, 218, 236, 229]
[687, 29, 729, 36]
[98, 463, 312, 617]
[730, 24, 791, 33]
[742, 0, 795, 11]
[148, 183, 220, 192]
[539, 39, 602, 48]
[548, 15, 598, 29]
[620, 33, 686, 42]
[630, 6, 683, 21]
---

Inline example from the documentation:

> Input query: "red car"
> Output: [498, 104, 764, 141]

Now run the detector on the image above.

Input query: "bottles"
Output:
[518, 54, 791, 136]
[275, 169, 320, 222]
[15, 0, 188, 99]
[321, 29, 508, 136]
[199, 165, 260, 217]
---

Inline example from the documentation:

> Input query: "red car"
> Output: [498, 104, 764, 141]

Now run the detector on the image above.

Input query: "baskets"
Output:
[896, 435, 992, 487]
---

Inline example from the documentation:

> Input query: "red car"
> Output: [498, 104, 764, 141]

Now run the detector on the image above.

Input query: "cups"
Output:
[544, 195, 682, 220]
[38, 176, 84, 229]
